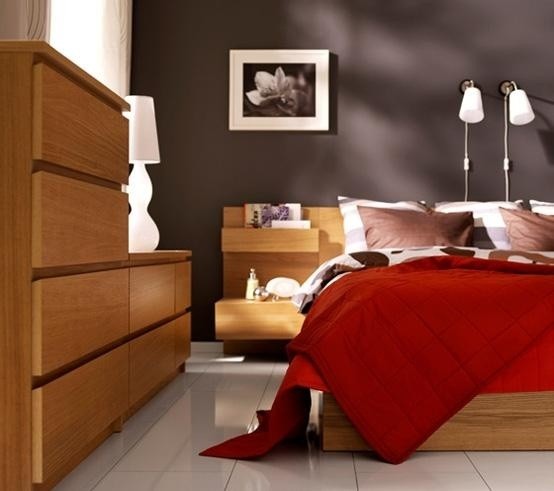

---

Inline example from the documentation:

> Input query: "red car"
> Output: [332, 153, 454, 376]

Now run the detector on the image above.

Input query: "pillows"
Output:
[336, 195, 554, 254]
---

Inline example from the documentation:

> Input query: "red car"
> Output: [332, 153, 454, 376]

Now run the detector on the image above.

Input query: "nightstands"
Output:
[214, 206, 320, 359]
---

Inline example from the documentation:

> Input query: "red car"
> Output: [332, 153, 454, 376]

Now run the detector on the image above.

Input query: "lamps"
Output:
[119, 96, 162, 253]
[456, 79, 484, 124]
[498, 79, 534, 128]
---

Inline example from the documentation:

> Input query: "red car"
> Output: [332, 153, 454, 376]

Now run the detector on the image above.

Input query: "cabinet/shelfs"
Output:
[1, 40, 193, 491]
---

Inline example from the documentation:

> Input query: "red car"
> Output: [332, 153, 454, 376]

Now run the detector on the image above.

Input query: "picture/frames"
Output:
[226, 48, 330, 132]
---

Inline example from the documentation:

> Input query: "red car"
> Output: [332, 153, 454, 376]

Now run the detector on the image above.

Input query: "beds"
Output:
[320, 254, 554, 450]
